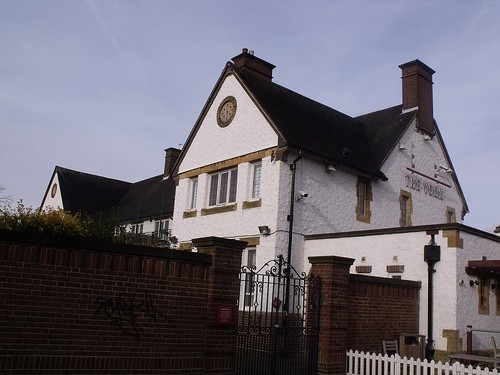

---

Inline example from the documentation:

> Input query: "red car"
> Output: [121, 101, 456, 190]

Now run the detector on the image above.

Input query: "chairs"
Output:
[383, 340, 398, 354]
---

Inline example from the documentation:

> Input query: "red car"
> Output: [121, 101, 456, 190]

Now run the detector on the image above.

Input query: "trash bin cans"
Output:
[398, 333, 425, 362]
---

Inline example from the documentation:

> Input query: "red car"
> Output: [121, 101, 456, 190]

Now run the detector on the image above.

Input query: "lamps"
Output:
[327, 165, 336, 176]
[423, 135, 431, 141]
[258, 226, 270, 235]
[446, 166, 453, 174]
[399, 144, 408, 152]
